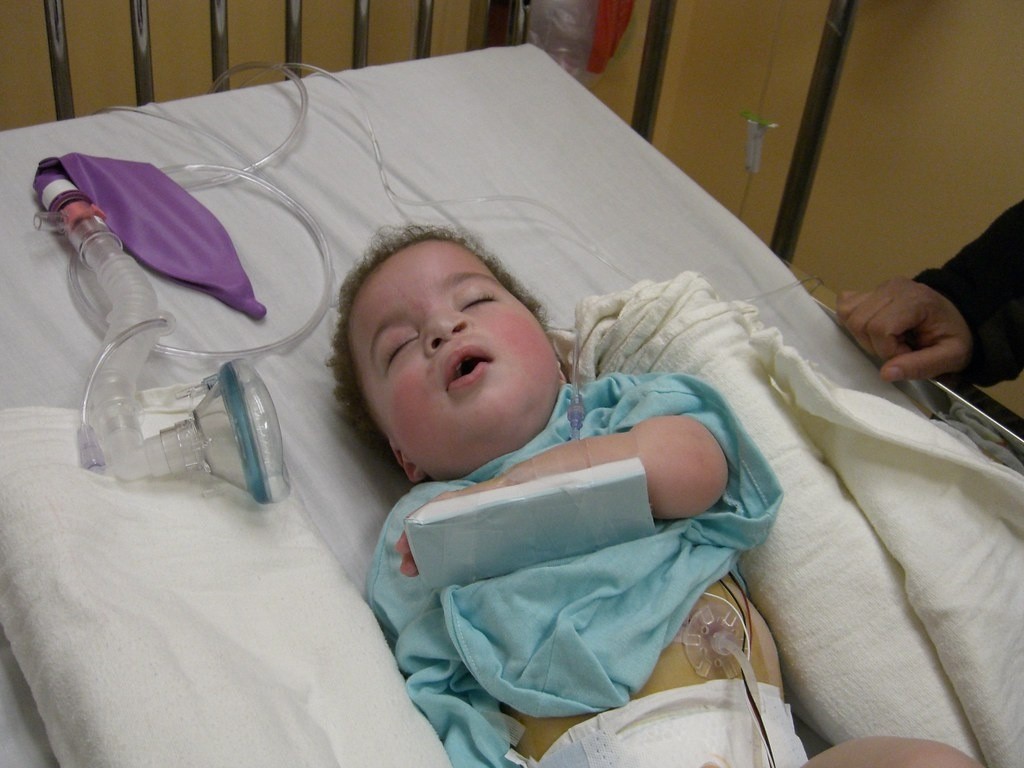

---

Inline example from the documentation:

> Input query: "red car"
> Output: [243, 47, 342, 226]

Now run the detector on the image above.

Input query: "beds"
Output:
[0, 0, 1024, 768]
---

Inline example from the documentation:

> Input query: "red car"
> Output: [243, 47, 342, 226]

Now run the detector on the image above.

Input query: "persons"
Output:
[840, 200, 1024, 386]
[328, 228, 984, 767]
